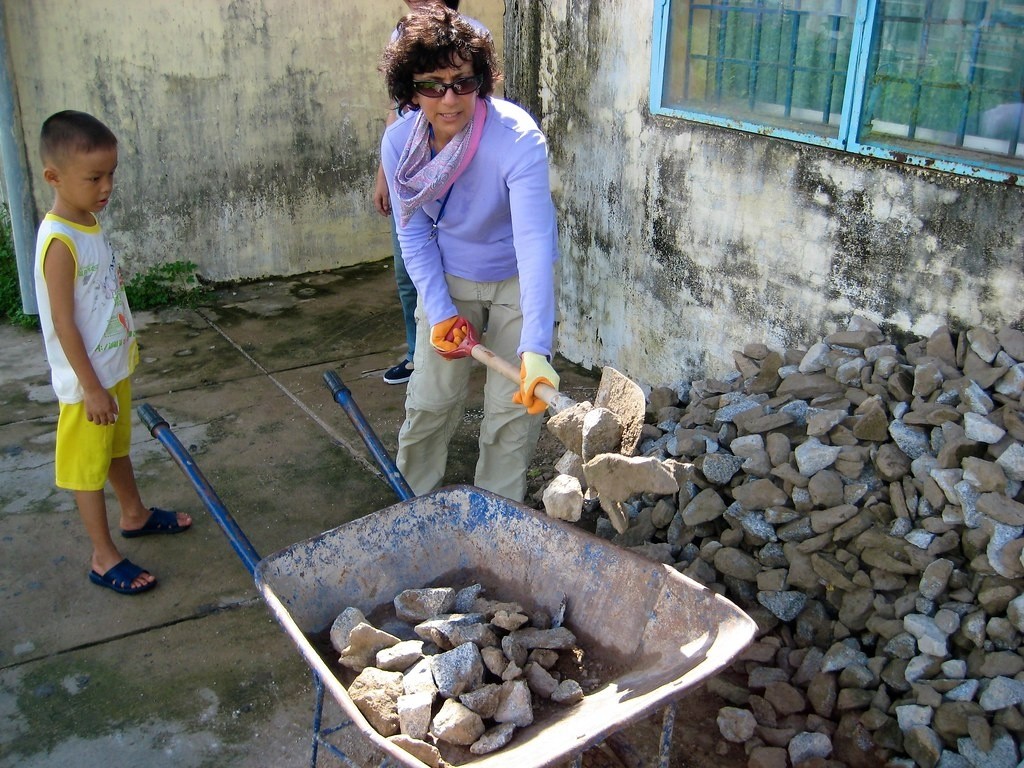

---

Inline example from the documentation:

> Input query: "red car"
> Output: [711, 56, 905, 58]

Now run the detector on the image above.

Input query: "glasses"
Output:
[407, 69, 485, 98]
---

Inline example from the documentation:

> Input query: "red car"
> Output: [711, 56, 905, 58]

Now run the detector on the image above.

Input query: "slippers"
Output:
[88, 556, 158, 594]
[121, 508, 190, 537]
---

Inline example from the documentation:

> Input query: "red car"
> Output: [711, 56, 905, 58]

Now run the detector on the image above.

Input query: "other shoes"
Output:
[382, 359, 415, 384]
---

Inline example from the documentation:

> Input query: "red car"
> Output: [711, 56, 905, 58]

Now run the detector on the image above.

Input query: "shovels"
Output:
[433, 316, 647, 460]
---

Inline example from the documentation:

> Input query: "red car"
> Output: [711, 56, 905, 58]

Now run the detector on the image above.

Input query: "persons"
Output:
[381, 6, 560, 498]
[34, 110, 193, 595]
[954, 102, 1024, 144]
[374, 0, 461, 385]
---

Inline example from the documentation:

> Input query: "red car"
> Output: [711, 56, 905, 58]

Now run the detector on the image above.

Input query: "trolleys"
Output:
[139, 368, 761, 768]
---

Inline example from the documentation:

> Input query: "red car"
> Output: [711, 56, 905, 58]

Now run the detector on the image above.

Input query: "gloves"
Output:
[429, 316, 467, 362]
[511, 351, 560, 414]
